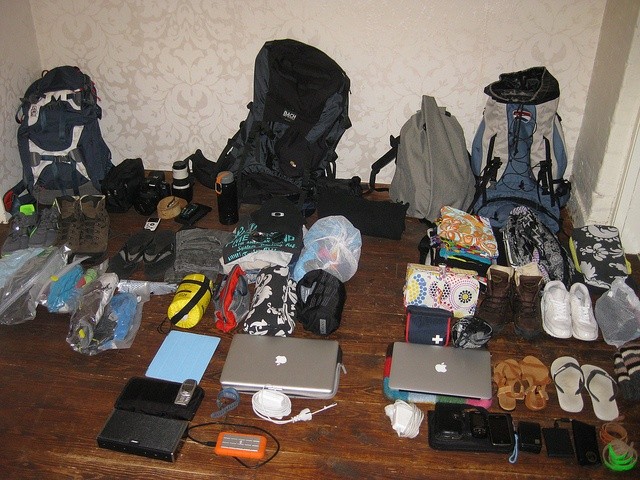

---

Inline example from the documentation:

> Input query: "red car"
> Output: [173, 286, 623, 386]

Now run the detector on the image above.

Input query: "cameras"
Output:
[132, 170, 168, 217]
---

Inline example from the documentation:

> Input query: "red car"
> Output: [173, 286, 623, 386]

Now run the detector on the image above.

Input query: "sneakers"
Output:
[570, 282, 599, 342]
[2, 204, 39, 257]
[28, 200, 61, 247]
[540, 279, 572, 339]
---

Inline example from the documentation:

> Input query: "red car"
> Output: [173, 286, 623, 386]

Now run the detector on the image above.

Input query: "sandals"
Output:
[493, 358, 525, 412]
[519, 355, 551, 411]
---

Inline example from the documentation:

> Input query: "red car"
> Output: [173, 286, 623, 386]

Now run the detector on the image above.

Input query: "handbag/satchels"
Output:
[243, 265, 296, 337]
[101, 158, 145, 211]
[295, 269, 347, 337]
[405, 306, 453, 347]
[427, 410, 519, 464]
[213, 264, 252, 333]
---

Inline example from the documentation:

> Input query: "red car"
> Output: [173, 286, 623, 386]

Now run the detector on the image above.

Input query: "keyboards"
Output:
[212, 431, 267, 460]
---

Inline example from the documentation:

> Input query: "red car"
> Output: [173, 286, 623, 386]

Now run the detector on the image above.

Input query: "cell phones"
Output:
[542, 428, 575, 458]
[469, 408, 488, 440]
[484, 411, 514, 449]
[173, 379, 197, 406]
[570, 419, 601, 466]
[433, 404, 466, 436]
[143, 215, 163, 231]
[517, 421, 543, 453]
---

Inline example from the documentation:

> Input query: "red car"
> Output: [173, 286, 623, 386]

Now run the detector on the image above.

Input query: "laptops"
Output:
[387, 341, 493, 400]
[220, 333, 339, 397]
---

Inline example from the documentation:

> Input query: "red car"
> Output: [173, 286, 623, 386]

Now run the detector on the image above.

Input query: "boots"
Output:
[476, 264, 514, 331]
[78, 194, 111, 268]
[514, 261, 543, 340]
[51, 196, 81, 253]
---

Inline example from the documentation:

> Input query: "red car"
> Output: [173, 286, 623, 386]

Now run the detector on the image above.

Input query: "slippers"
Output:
[580, 364, 620, 421]
[551, 355, 585, 414]
[107, 230, 154, 279]
[143, 231, 176, 280]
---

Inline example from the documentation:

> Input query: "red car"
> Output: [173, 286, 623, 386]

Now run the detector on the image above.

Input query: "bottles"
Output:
[215, 171, 239, 225]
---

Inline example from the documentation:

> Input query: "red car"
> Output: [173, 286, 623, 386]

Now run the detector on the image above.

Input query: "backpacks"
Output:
[362, 95, 476, 225]
[184, 40, 352, 219]
[2, 66, 114, 213]
[468, 66, 572, 235]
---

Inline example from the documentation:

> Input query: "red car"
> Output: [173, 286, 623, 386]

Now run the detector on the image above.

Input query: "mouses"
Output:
[177, 201, 200, 221]
